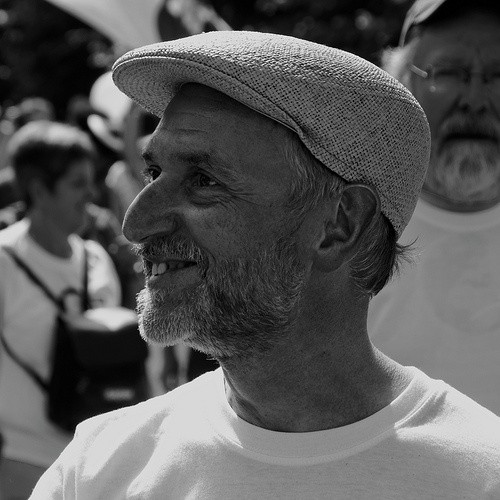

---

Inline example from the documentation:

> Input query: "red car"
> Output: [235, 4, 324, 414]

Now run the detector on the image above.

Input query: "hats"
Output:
[402, 0, 500, 45]
[111, 30, 431, 238]
[87, 113, 126, 155]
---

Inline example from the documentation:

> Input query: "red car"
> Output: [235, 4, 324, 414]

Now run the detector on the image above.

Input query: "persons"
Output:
[0, 116, 124, 500]
[19, 28, 499, 500]
[365, 0, 498, 416]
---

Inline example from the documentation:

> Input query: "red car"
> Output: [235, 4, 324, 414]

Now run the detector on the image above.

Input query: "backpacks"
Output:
[0, 241, 150, 434]
